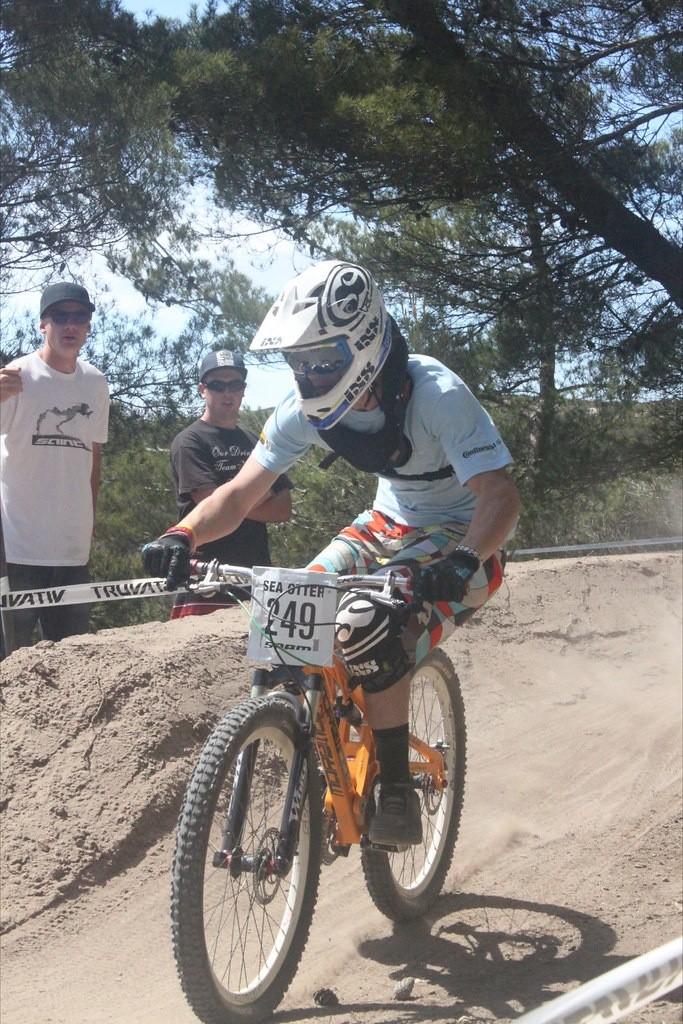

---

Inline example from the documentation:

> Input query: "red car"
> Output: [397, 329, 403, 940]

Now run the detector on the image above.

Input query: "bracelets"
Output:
[455, 545, 482, 566]
[166, 523, 197, 558]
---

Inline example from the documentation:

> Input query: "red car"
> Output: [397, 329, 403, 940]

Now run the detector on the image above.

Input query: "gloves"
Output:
[417, 545, 479, 603]
[142, 527, 196, 592]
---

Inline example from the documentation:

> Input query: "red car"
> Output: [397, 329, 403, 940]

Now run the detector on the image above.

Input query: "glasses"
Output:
[201, 380, 246, 392]
[294, 360, 343, 376]
[45, 310, 92, 324]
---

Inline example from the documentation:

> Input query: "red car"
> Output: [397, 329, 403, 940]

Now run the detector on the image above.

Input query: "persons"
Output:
[144, 257, 521, 845]
[0, 282, 109, 661]
[169, 349, 294, 621]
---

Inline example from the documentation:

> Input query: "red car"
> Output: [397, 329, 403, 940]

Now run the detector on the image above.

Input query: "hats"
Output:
[198, 349, 248, 384]
[40, 282, 95, 319]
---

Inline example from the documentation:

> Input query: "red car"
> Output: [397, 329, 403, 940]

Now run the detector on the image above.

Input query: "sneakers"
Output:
[367, 775, 423, 844]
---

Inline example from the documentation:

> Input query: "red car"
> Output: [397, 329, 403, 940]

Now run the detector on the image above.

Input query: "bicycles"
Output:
[169, 559, 468, 1023]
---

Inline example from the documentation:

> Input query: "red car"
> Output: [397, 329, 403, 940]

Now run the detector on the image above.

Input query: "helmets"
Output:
[250, 260, 400, 431]
